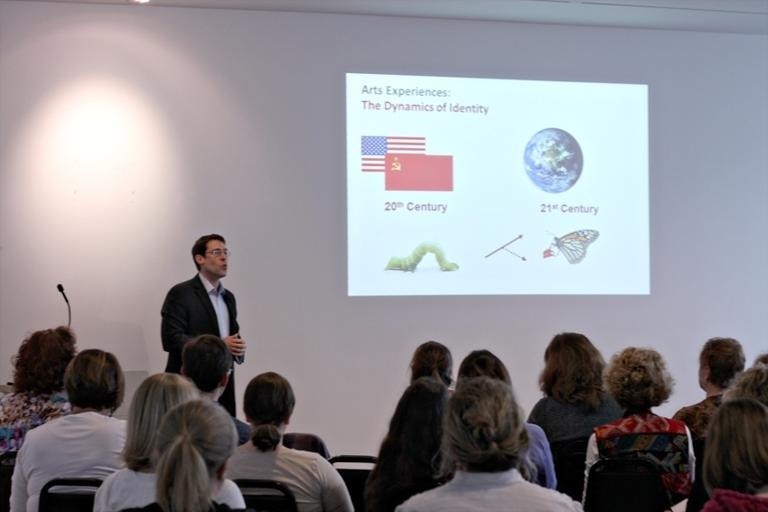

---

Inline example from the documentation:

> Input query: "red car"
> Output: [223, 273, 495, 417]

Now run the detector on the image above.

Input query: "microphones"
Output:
[57, 283, 71, 329]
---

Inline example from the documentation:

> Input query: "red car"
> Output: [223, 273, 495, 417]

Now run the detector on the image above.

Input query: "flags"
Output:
[360, 134, 426, 174]
[381, 152, 456, 193]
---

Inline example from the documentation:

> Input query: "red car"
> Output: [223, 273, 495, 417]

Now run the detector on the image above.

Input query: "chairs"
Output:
[231, 478, 299, 512]
[583, 455, 672, 510]
[38, 478, 104, 512]
[329, 455, 380, 512]
[0, 451, 17, 512]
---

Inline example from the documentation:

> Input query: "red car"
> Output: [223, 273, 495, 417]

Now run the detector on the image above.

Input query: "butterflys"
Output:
[548, 228, 598, 265]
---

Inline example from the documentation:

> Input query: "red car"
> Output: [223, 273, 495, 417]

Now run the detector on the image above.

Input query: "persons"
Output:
[581, 345, 699, 511]
[0, 324, 82, 467]
[8, 348, 131, 512]
[363, 376, 457, 512]
[391, 374, 585, 512]
[407, 339, 457, 398]
[179, 334, 254, 447]
[91, 371, 248, 511]
[669, 335, 746, 511]
[116, 397, 255, 511]
[719, 363, 768, 407]
[697, 395, 767, 511]
[160, 233, 248, 421]
[220, 370, 355, 511]
[456, 348, 561, 490]
[527, 331, 627, 503]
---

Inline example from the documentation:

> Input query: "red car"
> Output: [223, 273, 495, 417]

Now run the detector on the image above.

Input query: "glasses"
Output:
[203, 249, 231, 258]
